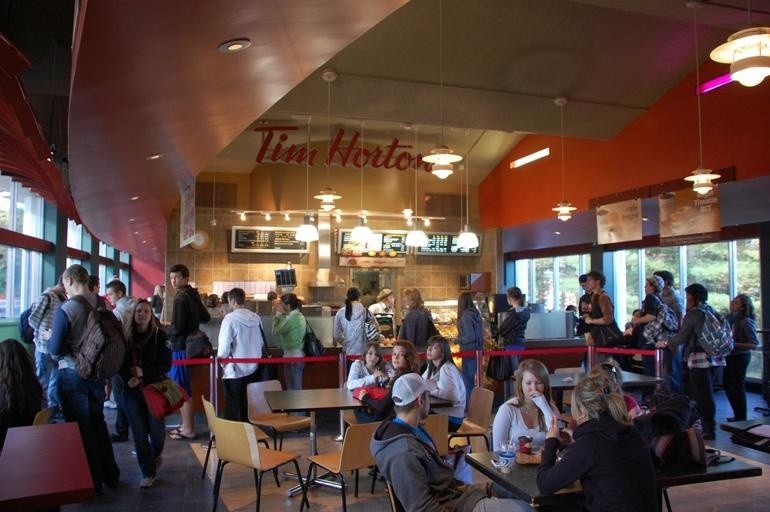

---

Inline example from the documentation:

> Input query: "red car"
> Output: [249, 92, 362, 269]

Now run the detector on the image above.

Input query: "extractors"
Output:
[305, 214, 343, 290]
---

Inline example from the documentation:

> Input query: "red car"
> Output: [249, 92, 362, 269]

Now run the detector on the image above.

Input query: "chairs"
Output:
[32, 404, 57, 426]
[186, 364, 769, 512]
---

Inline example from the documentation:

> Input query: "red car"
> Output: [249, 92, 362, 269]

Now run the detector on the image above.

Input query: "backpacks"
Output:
[643, 294, 678, 346]
[70, 296, 126, 383]
[18, 291, 52, 344]
[698, 305, 734, 359]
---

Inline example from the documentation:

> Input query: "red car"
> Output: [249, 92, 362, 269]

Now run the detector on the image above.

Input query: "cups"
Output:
[498, 441, 517, 475]
[377, 375, 387, 387]
[659, 192, 676, 222]
[518, 435, 534, 454]
[606, 226, 618, 241]
[597, 209, 611, 229]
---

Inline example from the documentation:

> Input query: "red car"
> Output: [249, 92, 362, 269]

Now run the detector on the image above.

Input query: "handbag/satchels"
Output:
[185, 329, 213, 359]
[353, 384, 389, 405]
[591, 320, 622, 346]
[364, 308, 381, 342]
[486, 349, 512, 382]
[260, 335, 279, 380]
[634, 395, 708, 474]
[142, 378, 189, 419]
[304, 320, 325, 357]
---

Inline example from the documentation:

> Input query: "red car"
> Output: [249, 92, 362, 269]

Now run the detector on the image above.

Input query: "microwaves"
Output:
[457, 272, 490, 293]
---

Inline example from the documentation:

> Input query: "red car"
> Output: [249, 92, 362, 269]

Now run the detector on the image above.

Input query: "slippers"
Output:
[170, 428, 198, 439]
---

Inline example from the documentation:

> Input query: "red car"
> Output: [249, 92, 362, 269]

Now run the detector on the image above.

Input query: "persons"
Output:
[201, 287, 307, 422]
[332, 285, 530, 433]
[0, 340, 42, 453]
[28, 264, 210, 486]
[370, 357, 662, 512]
[567, 270, 759, 441]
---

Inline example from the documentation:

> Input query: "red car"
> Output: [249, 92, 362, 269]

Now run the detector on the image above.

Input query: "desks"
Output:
[0, 420, 99, 512]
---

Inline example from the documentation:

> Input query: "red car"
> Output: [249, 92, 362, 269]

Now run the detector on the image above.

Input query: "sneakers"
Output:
[109, 432, 130, 443]
[727, 416, 740, 421]
[703, 433, 716, 440]
[155, 456, 161, 469]
[140, 475, 159, 488]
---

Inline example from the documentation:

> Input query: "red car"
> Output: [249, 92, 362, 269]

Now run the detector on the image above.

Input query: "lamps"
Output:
[549, 95, 578, 224]
[226, 205, 448, 228]
[705, 1, 770, 90]
[291, 66, 483, 256]
[680, 2, 723, 196]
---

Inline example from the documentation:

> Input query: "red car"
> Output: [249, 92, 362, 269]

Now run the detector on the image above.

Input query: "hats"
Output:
[378, 288, 394, 299]
[391, 373, 437, 406]
[646, 275, 665, 292]
[684, 282, 709, 301]
